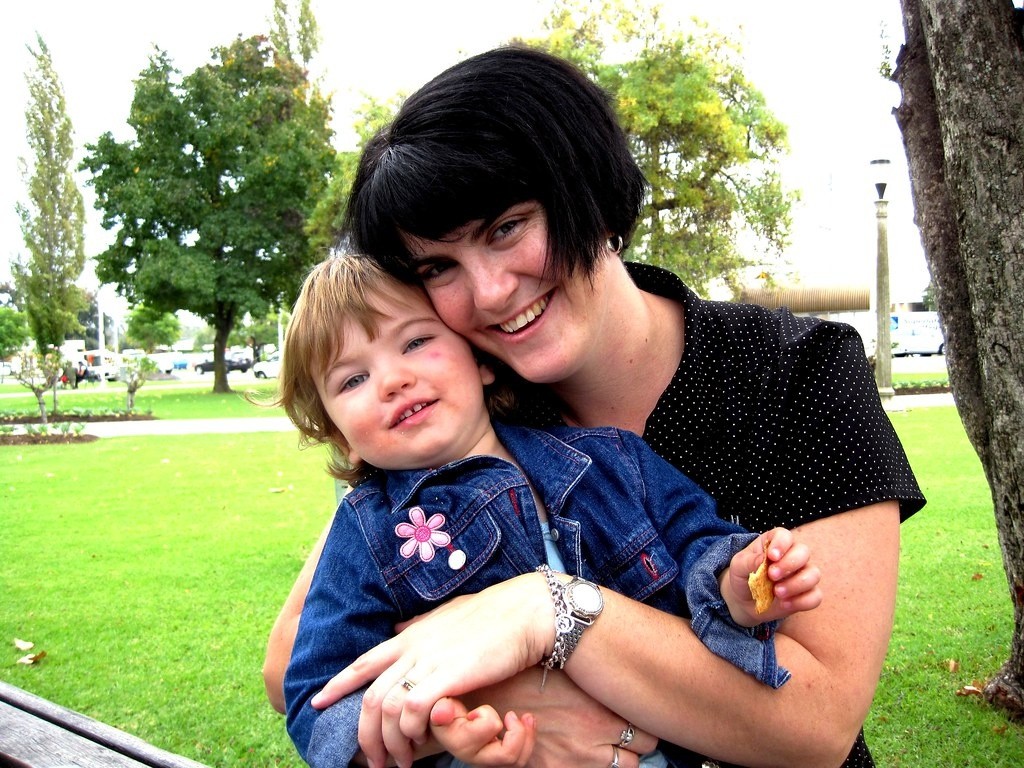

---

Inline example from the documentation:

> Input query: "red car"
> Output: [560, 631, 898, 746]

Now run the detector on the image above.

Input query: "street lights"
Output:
[870, 144, 896, 399]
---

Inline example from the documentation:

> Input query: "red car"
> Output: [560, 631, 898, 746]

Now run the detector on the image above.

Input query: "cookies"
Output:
[748, 540, 776, 616]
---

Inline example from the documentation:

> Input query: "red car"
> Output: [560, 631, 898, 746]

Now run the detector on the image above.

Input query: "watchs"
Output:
[560, 571, 604, 659]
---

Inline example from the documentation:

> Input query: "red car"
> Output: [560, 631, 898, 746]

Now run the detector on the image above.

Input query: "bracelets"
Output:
[534, 558, 575, 693]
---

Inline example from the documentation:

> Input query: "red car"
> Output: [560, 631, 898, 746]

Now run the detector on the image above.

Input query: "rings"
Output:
[611, 745, 619, 768]
[619, 724, 635, 748]
[399, 677, 415, 691]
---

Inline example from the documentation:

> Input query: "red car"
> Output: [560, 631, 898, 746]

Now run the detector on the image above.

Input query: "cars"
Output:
[194, 349, 251, 374]
[253, 352, 280, 378]
[172, 358, 188, 369]
[105, 363, 120, 381]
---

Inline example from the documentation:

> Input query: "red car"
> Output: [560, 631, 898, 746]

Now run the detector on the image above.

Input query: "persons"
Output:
[261, 44, 928, 768]
[258, 253, 822, 768]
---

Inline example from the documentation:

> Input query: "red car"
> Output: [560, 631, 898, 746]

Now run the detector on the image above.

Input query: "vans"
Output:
[884, 311, 947, 356]
